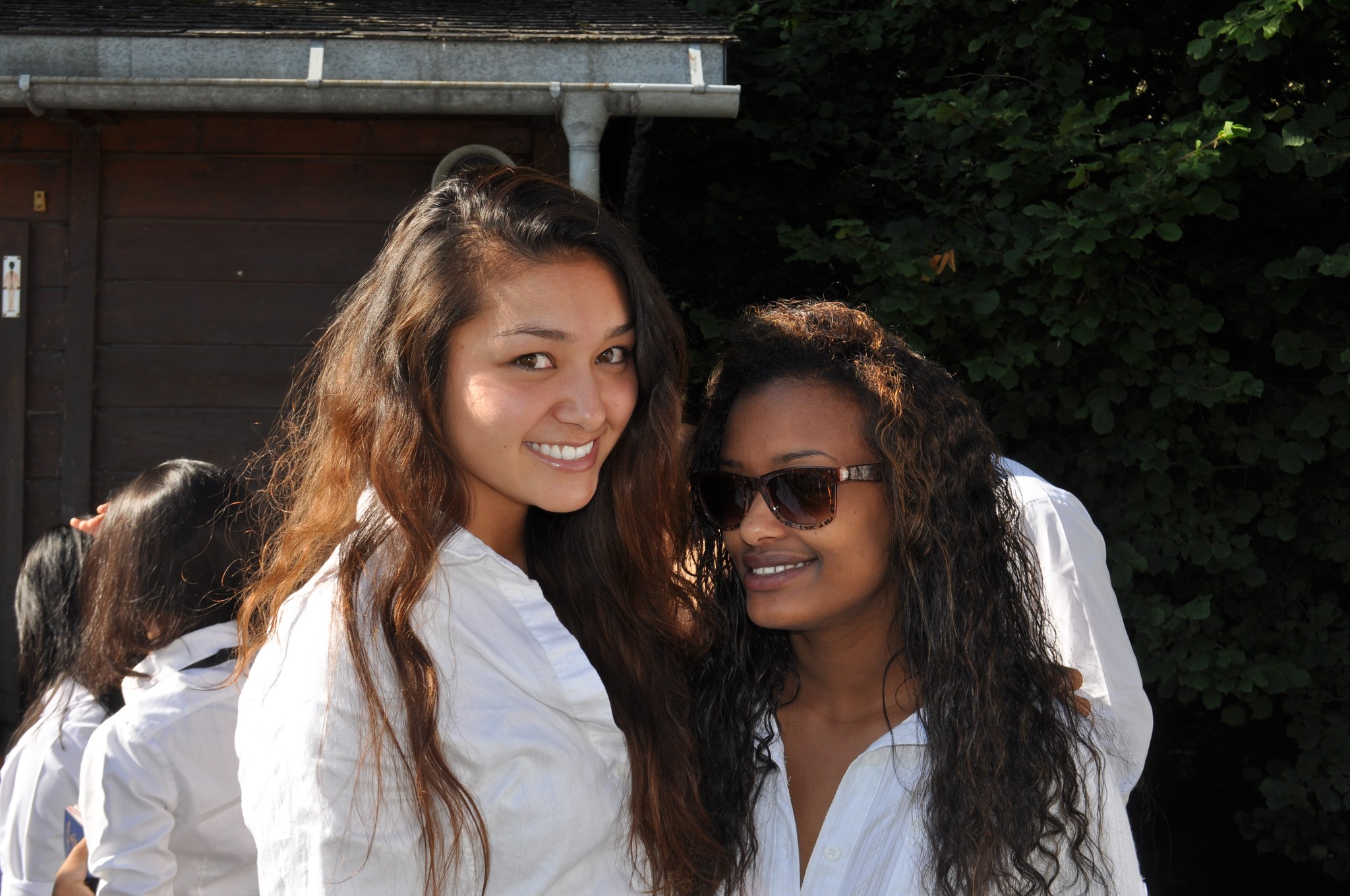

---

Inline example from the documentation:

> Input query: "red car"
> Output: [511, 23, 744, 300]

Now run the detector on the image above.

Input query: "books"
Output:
[64, 804, 99, 892]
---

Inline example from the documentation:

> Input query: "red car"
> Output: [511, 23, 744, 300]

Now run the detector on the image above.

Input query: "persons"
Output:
[988, 449, 1153, 806]
[48, 458, 275, 896]
[685, 301, 1148, 896]
[0, 525, 142, 896]
[221, 164, 1092, 896]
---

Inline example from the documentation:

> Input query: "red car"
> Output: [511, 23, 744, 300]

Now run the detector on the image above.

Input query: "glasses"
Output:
[689, 464, 889, 532]
